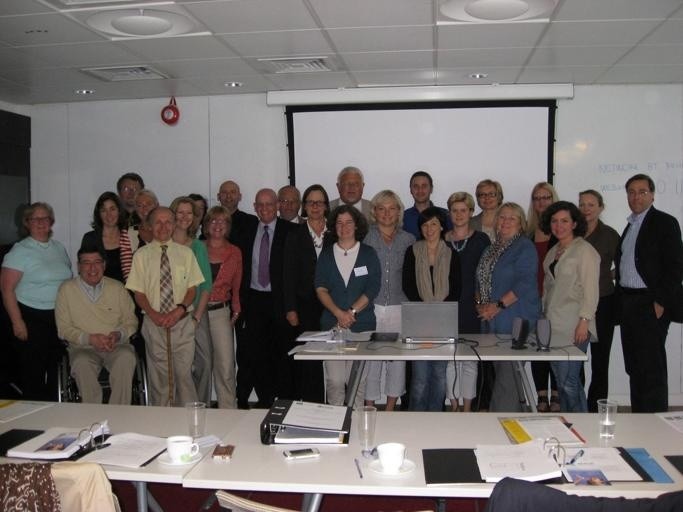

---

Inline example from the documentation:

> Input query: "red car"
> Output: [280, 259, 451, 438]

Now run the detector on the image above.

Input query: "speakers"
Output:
[511, 316, 529, 350]
[537, 319, 552, 353]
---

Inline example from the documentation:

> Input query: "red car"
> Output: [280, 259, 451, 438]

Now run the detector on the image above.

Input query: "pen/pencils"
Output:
[354, 459, 363, 478]
[570, 450, 583, 464]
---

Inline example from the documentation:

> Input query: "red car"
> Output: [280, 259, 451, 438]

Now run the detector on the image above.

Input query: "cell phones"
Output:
[283, 448, 319, 459]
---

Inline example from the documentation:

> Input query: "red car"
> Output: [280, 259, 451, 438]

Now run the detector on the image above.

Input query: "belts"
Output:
[207, 300, 230, 310]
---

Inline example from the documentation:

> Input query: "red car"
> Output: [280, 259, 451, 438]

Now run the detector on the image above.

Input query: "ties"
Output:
[160, 245, 174, 313]
[258, 226, 270, 288]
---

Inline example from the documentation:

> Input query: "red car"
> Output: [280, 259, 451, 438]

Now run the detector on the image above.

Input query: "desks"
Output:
[0, 392, 247, 512]
[292, 330, 590, 413]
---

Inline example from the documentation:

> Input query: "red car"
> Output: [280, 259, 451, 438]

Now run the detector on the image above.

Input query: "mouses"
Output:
[380, 334, 390, 341]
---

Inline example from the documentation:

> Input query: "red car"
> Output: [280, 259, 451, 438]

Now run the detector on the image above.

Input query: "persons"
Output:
[0, 201, 74, 403]
[46, 443, 66, 453]
[571, 476, 606, 486]
[55, 166, 622, 419]
[620, 174, 683, 414]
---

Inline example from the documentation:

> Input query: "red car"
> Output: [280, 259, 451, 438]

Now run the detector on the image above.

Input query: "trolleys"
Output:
[55, 314, 150, 405]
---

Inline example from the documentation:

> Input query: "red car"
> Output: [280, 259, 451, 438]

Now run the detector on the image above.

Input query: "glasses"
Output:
[534, 195, 551, 200]
[308, 200, 325, 206]
[479, 192, 497, 197]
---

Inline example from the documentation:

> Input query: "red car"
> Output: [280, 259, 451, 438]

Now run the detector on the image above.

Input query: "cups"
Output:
[166, 436, 200, 462]
[335, 328, 347, 351]
[376, 442, 406, 471]
[356, 406, 378, 451]
[597, 398, 618, 442]
[186, 401, 206, 438]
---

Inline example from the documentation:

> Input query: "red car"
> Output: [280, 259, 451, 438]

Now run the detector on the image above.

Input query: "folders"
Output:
[260, 399, 352, 444]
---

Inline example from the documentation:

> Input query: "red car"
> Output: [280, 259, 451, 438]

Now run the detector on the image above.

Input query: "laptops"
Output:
[401, 301, 459, 344]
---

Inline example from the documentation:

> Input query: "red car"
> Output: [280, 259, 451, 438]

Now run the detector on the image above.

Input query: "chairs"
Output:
[0, 461, 121, 512]
[486, 477, 683, 511]
[214, 490, 300, 512]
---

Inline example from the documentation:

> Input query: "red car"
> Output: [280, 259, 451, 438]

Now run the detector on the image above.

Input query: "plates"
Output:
[368, 459, 416, 475]
[158, 452, 203, 467]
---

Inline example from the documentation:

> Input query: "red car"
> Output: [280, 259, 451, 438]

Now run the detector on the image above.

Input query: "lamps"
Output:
[57, 1, 215, 43]
[434, 0, 553, 26]
